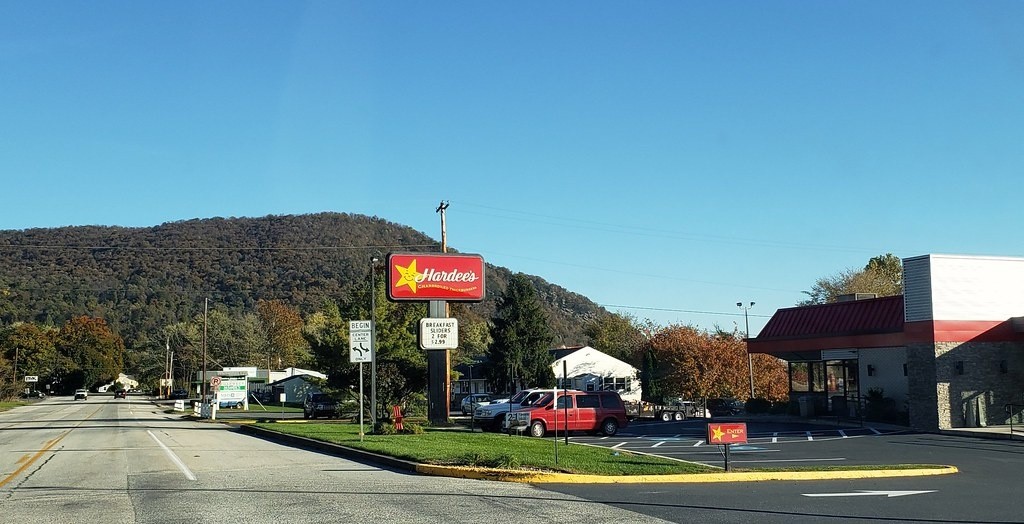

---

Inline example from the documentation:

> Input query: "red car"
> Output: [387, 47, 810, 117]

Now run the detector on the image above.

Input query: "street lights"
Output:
[737, 302, 755, 400]
[370, 255, 380, 434]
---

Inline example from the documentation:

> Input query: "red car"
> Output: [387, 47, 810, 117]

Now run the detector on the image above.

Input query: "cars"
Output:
[20, 391, 45, 400]
[74, 389, 87, 401]
[114, 390, 126, 399]
[169, 390, 188, 399]
[461, 393, 513, 416]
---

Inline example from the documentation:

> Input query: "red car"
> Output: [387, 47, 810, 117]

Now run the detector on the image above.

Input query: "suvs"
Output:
[474, 387, 567, 433]
[304, 391, 335, 420]
[501, 390, 628, 438]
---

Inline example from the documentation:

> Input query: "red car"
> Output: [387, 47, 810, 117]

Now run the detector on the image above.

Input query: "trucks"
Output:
[654, 401, 696, 422]
